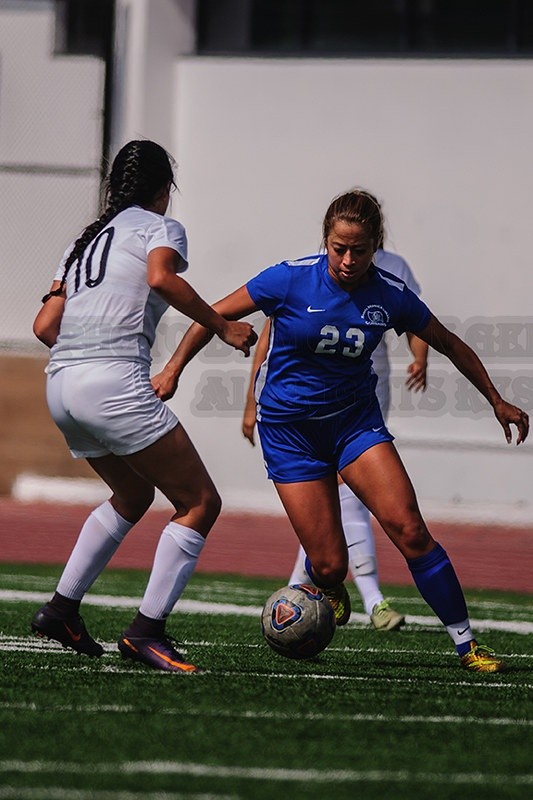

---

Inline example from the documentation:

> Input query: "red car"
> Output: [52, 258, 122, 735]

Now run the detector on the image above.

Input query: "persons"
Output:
[29, 141, 259, 672]
[149, 189, 531, 672]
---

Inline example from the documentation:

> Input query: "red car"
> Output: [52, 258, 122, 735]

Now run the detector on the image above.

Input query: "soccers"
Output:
[262, 585, 336, 658]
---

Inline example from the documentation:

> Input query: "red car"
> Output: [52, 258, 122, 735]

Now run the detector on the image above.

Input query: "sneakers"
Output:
[31, 603, 104, 659]
[460, 641, 506, 674]
[370, 600, 404, 632]
[305, 557, 351, 626]
[118, 632, 197, 674]
